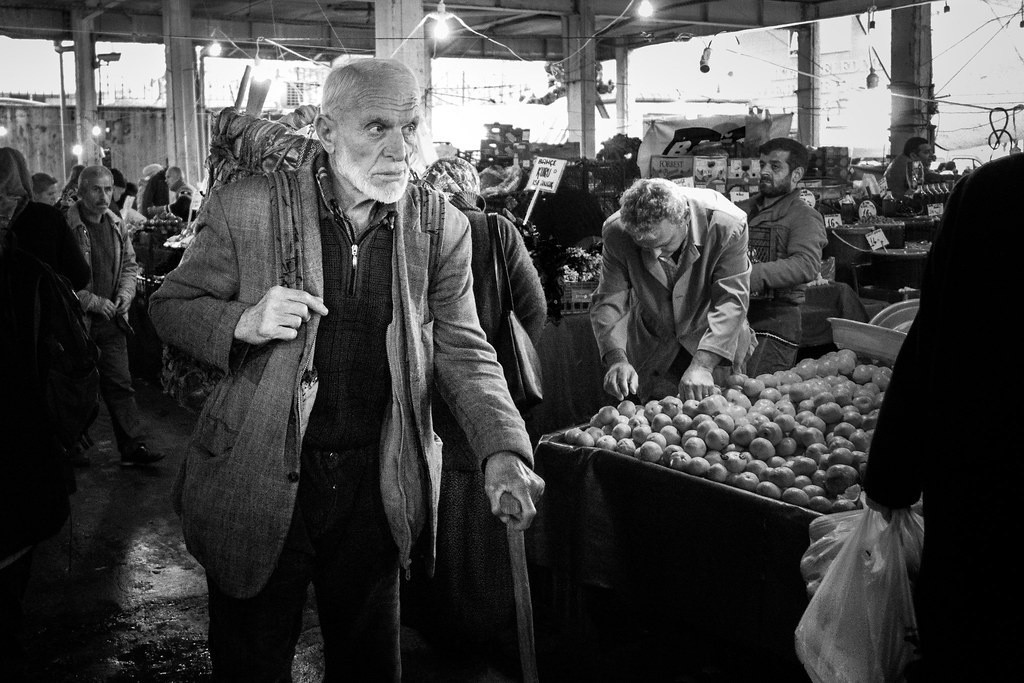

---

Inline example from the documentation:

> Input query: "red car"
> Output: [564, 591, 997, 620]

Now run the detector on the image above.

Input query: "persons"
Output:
[147, 57, 545, 680]
[30, 173, 57, 205]
[0, 148, 93, 495]
[881, 137, 934, 194]
[865, 152, 1023, 683]
[590, 176, 758, 407]
[147, 166, 203, 223]
[105, 158, 170, 229]
[712, 137, 829, 385]
[416, 155, 549, 577]
[54, 165, 84, 214]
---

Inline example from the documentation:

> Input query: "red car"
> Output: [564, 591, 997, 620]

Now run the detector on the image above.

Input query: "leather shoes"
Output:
[123, 446, 167, 468]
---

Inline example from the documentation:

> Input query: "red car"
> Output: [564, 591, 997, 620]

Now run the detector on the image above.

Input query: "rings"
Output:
[702, 394, 709, 397]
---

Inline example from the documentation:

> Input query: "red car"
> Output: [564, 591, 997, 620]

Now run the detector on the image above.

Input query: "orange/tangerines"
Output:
[563, 349, 893, 514]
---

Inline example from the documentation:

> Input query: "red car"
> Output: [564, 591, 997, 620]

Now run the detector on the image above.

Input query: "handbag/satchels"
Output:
[501, 309, 544, 408]
[797, 497, 918, 683]
[800, 505, 923, 599]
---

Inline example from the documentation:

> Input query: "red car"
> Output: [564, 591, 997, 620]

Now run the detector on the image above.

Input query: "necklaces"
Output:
[64, 166, 167, 471]
